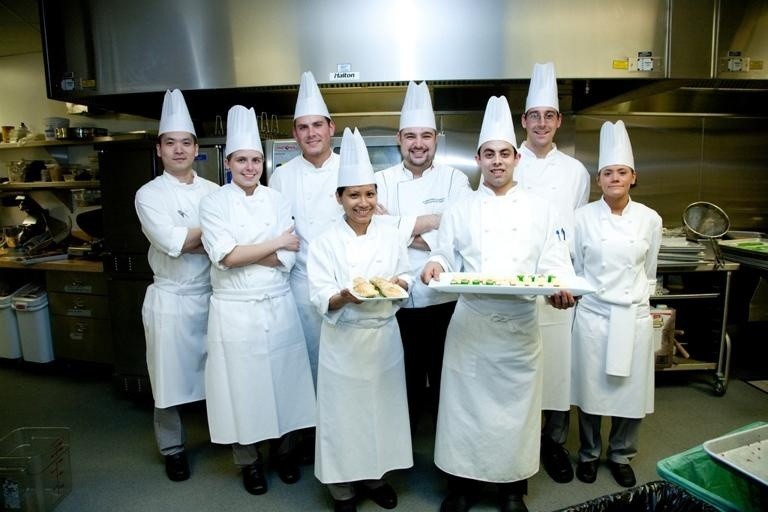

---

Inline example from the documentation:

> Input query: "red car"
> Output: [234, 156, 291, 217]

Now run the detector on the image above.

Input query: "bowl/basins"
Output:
[67, 127, 109, 140]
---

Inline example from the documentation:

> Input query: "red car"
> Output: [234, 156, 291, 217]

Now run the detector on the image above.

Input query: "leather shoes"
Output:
[276, 450, 299, 485]
[372, 482, 397, 509]
[164, 456, 189, 483]
[577, 456, 599, 484]
[607, 458, 635, 487]
[541, 436, 574, 484]
[242, 463, 267, 495]
[498, 489, 528, 512]
[441, 487, 475, 512]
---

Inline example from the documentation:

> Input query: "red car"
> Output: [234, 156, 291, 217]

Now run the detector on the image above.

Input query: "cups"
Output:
[45, 164, 61, 182]
[55, 127, 67, 138]
[2, 126, 15, 143]
[8, 160, 27, 184]
[63, 175, 75, 181]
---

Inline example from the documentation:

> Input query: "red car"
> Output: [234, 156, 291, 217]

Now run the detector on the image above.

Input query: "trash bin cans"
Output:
[547, 420, 768, 512]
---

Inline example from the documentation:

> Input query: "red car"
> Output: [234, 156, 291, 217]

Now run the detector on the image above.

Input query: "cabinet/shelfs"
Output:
[0, 138, 107, 193]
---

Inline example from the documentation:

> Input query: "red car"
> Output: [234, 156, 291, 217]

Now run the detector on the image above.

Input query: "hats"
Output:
[225, 105, 264, 164]
[525, 62, 560, 113]
[399, 81, 436, 131]
[337, 126, 376, 187]
[598, 120, 634, 174]
[158, 88, 197, 137]
[293, 71, 329, 120]
[477, 96, 517, 154]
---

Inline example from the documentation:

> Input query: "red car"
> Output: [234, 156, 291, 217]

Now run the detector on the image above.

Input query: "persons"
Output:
[134, 132, 220, 482]
[572, 163, 663, 487]
[199, 150, 316, 496]
[512, 107, 592, 484]
[269, 115, 341, 461]
[372, 127, 472, 436]
[307, 183, 416, 512]
[419, 140, 582, 512]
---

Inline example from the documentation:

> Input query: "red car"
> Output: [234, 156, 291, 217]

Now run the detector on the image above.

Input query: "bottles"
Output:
[16, 122, 28, 139]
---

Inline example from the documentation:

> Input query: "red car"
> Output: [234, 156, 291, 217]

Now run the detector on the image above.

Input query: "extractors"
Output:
[34, 0, 767, 115]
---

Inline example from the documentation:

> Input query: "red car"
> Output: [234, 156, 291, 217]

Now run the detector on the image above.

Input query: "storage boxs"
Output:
[0, 427, 72, 512]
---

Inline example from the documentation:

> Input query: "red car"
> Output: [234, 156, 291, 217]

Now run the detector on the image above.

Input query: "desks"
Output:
[0, 249, 109, 370]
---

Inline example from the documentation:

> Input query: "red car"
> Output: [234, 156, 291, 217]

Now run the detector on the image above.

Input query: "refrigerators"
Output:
[93, 133, 231, 404]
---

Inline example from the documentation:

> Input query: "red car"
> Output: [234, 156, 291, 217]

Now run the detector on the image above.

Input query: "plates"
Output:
[344, 282, 410, 302]
[43, 117, 70, 132]
[426, 270, 599, 295]
